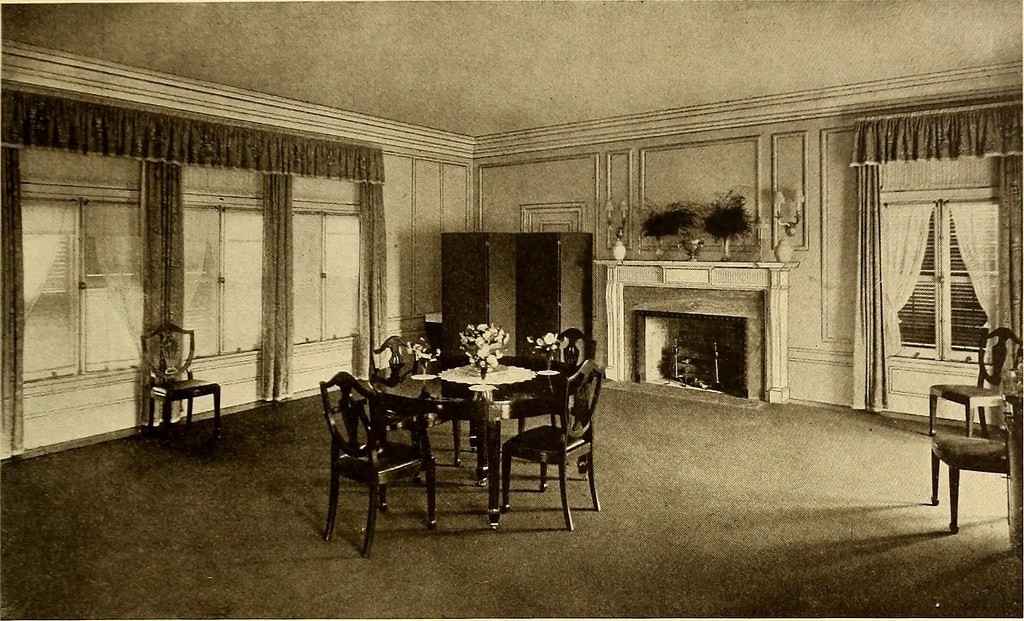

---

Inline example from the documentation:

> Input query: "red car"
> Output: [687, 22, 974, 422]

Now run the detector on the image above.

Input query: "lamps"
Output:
[773, 191, 805, 237]
[605, 200, 629, 240]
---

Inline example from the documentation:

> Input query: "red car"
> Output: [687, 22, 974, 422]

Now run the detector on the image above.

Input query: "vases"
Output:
[421, 362, 427, 377]
[655, 237, 664, 261]
[545, 357, 552, 372]
[721, 238, 733, 262]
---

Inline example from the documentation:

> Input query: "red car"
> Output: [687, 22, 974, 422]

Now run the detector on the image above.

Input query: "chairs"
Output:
[320, 328, 604, 558]
[140, 322, 221, 444]
[931, 435, 1008, 534]
[929, 326, 1024, 436]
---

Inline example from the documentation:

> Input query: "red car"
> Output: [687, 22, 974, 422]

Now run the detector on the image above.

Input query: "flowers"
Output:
[405, 337, 440, 369]
[526, 333, 562, 362]
[460, 323, 511, 380]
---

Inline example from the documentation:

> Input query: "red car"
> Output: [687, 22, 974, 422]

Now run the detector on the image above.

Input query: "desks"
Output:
[368, 356, 594, 530]
